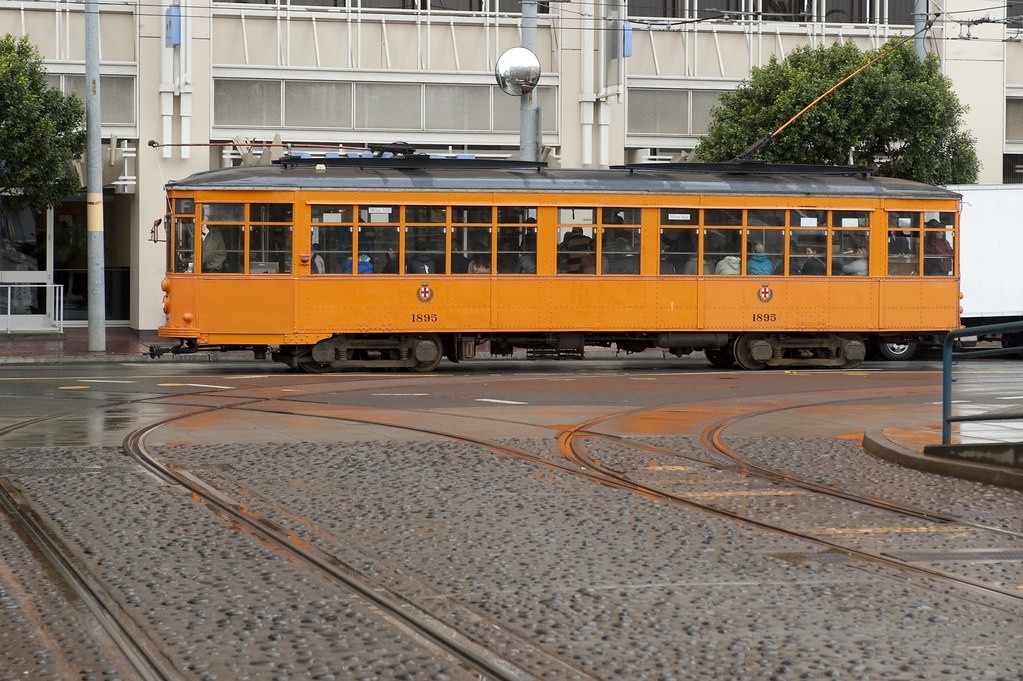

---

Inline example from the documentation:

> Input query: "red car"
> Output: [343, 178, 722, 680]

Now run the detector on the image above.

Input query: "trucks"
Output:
[878, 181, 1023, 361]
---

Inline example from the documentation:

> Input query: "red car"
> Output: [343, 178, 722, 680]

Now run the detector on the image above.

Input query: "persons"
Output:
[193, 220, 953, 274]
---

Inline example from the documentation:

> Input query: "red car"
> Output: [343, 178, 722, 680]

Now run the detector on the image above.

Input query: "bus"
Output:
[139, 12, 964, 376]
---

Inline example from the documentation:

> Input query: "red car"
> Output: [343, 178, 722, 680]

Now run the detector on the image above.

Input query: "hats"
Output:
[889, 211, 902, 218]
[924, 219, 938, 226]
